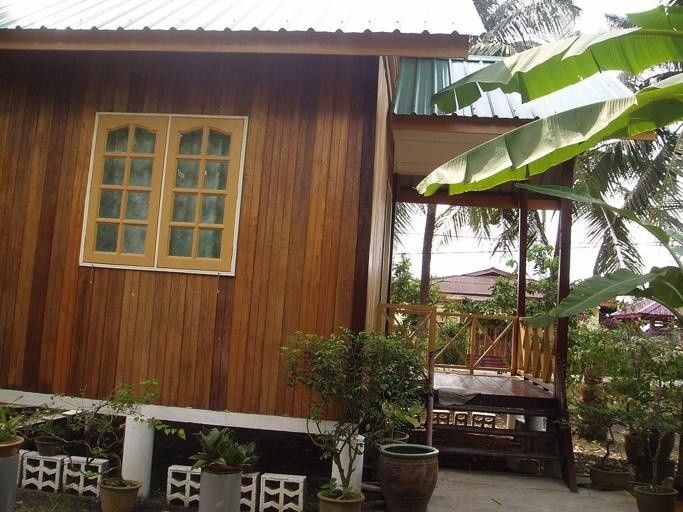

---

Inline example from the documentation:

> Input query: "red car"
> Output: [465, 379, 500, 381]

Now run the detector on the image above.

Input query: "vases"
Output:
[35, 433, 61, 457]
[376, 443, 441, 512]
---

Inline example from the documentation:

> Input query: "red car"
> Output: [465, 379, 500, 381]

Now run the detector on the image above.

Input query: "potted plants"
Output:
[602, 333, 683, 512]
[186, 423, 257, 512]
[546, 382, 634, 490]
[373, 397, 425, 452]
[0, 395, 31, 512]
[12, 374, 187, 512]
[276, 323, 433, 511]
